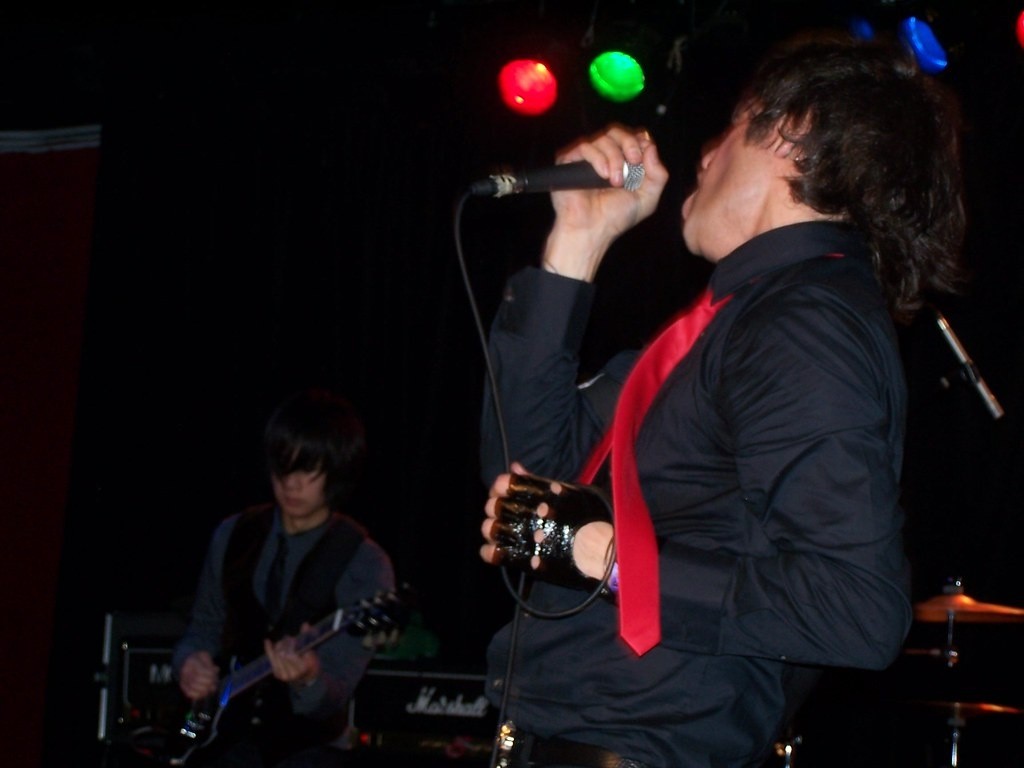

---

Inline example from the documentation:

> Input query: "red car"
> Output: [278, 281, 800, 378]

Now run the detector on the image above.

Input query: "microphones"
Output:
[471, 160, 645, 198]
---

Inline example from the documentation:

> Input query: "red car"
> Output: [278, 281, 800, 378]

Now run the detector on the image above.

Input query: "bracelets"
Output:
[609, 562, 618, 592]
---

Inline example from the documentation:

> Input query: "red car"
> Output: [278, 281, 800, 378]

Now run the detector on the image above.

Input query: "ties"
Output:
[261, 533, 290, 603]
[584, 290, 732, 655]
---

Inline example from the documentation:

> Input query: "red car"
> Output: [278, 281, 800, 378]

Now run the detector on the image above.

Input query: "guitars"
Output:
[167, 582, 398, 767]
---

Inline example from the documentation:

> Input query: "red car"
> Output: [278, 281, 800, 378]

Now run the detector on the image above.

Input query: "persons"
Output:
[479, 32, 963, 768]
[171, 390, 398, 768]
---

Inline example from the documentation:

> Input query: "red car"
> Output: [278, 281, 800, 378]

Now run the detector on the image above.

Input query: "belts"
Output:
[510, 733, 621, 768]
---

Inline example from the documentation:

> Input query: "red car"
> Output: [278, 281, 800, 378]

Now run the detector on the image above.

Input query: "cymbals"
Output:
[913, 594, 1024, 623]
[922, 699, 1021, 714]
